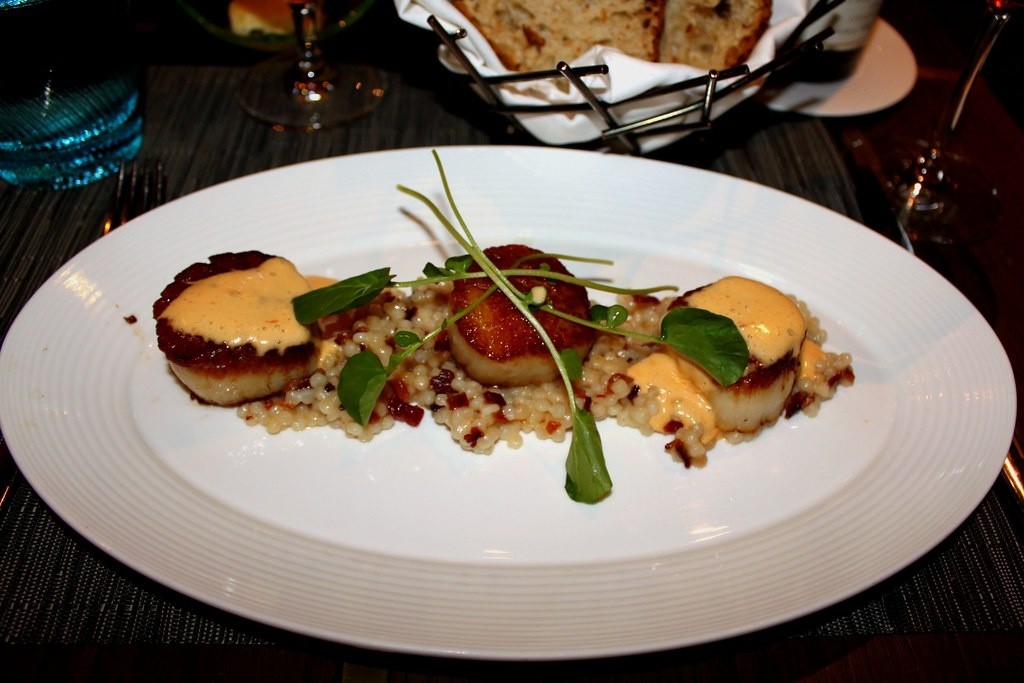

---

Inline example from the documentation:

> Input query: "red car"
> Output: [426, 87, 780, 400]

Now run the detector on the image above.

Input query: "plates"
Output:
[0, 144, 1018, 660]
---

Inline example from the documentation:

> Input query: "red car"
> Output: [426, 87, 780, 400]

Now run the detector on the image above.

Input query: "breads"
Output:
[444, 0, 773, 78]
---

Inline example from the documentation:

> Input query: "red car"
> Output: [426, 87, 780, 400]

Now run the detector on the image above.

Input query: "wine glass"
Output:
[857, 0, 1024, 246]
[238, 0, 382, 130]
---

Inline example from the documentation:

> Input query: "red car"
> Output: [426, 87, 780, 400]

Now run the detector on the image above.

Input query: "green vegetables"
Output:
[290, 147, 750, 507]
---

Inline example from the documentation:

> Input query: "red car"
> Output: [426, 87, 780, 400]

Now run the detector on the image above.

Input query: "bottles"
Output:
[759, 18, 918, 116]
[0, 0, 146, 191]
[796, 0, 880, 84]
